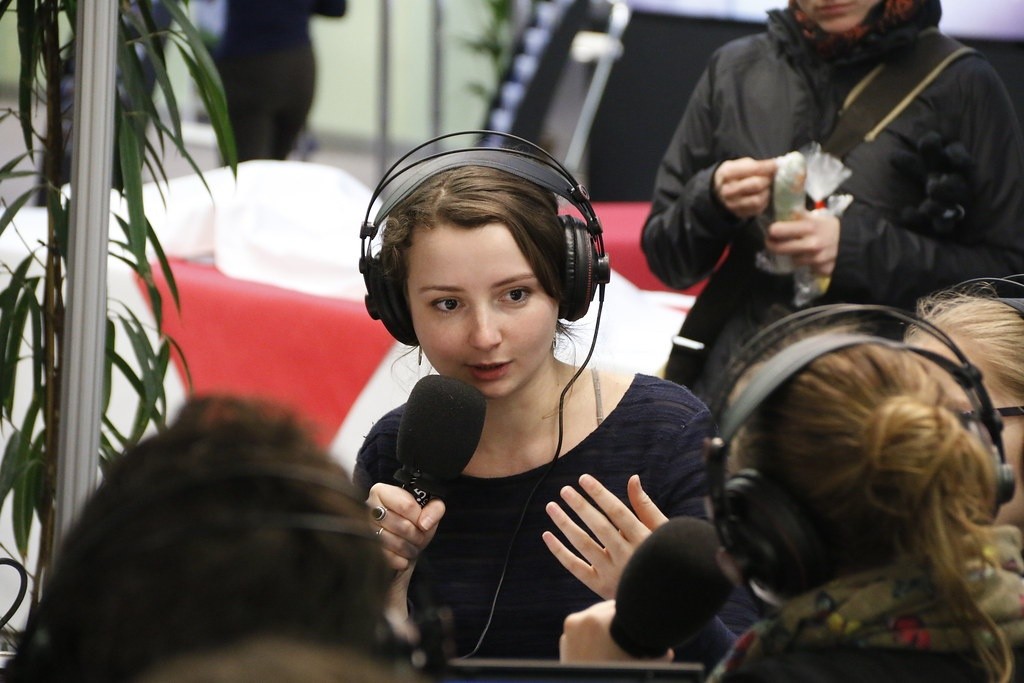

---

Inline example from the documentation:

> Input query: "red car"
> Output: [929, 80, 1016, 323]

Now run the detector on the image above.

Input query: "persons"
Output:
[213, 1, 349, 168]
[641, 1, 1024, 404]
[560, 276, 1024, 682]
[0, 394, 419, 682]
[350, 129, 760, 683]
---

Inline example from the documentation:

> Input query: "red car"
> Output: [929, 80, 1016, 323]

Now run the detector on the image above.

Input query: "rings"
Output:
[372, 506, 388, 521]
[376, 528, 384, 535]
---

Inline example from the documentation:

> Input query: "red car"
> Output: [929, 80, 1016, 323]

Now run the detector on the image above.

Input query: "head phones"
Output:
[689, 303, 1016, 611]
[359, 130, 611, 347]
[0, 462, 390, 683]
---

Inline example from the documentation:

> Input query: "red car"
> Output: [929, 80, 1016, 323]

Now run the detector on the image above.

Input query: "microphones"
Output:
[393, 373, 486, 509]
[611, 516, 738, 660]
